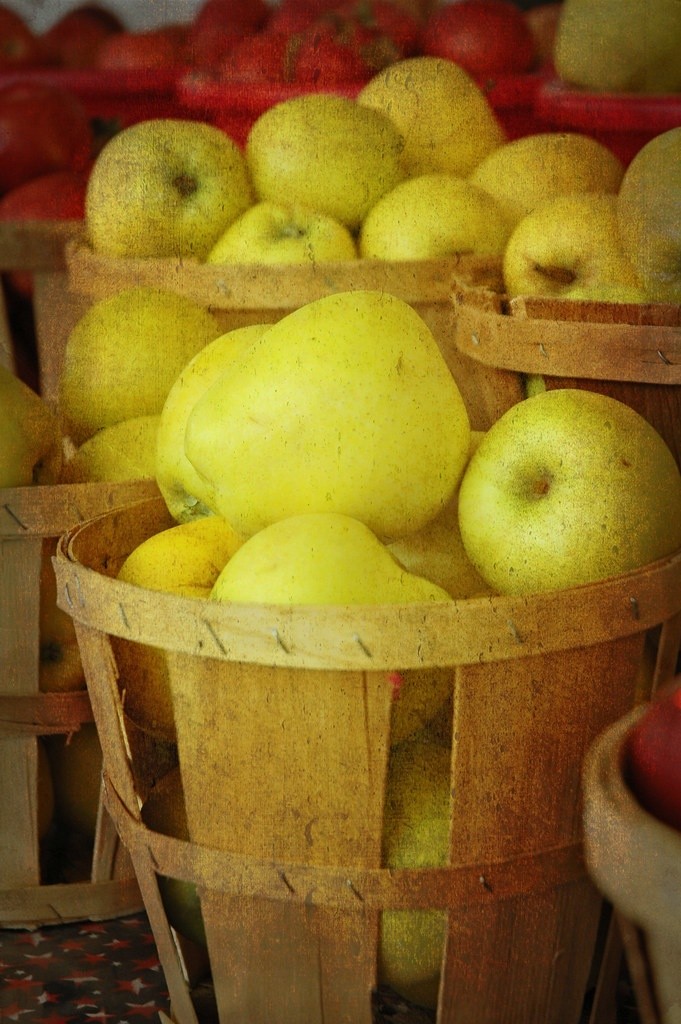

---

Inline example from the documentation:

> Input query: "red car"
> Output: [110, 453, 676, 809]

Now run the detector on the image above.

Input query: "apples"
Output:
[0, 0, 681, 1010]
